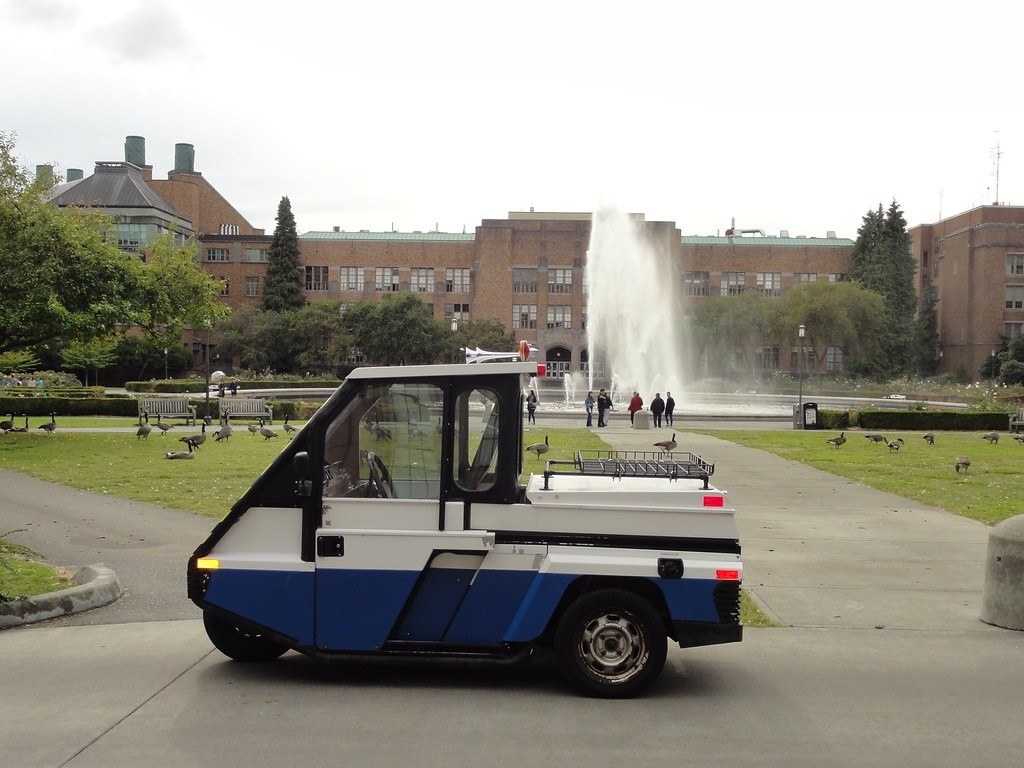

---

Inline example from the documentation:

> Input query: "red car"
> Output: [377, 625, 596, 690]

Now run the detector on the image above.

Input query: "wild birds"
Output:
[166, 439, 196, 459]
[282, 414, 301, 441]
[256, 416, 280, 441]
[1012, 436, 1024, 447]
[826, 432, 846, 449]
[364, 412, 392, 442]
[136, 411, 152, 440]
[406, 421, 428, 441]
[955, 454, 970, 472]
[0, 411, 17, 435]
[863, 434, 904, 453]
[652, 432, 678, 457]
[37, 408, 58, 434]
[246, 422, 260, 435]
[524, 432, 550, 459]
[13, 417, 29, 432]
[435, 416, 443, 438]
[155, 411, 174, 435]
[922, 431, 934, 446]
[211, 418, 232, 442]
[178, 420, 210, 450]
[981, 432, 999, 445]
[215, 411, 233, 442]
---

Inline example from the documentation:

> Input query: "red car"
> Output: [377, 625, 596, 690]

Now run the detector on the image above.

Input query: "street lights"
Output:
[216, 352, 220, 372]
[164, 345, 169, 381]
[991, 348, 996, 383]
[795, 322, 806, 429]
[203, 313, 212, 426]
[939, 348, 943, 391]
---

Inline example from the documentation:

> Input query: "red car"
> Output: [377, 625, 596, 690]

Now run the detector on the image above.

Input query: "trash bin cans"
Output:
[793, 402, 817, 430]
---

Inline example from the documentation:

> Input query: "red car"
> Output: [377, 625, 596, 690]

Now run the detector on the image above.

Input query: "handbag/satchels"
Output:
[589, 407, 592, 413]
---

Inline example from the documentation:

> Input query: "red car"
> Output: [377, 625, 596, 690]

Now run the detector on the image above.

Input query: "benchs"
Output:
[138, 398, 198, 425]
[219, 399, 273, 425]
[1008, 404, 1024, 434]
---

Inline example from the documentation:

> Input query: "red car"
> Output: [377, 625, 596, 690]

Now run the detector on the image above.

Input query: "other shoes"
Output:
[602, 424, 607, 426]
[598, 425, 603, 427]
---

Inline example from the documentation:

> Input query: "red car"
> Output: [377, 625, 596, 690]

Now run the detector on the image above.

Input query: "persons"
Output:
[11, 374, 44, 388]
[651, 393, 665, 428]
[218, 381, 226, 397]
[665, 392, 675, 428]
[598, 389, 643, 427]
[585, 391, 596, 428]
[230, 379, 238, 396]
[526, 390, 538, 425]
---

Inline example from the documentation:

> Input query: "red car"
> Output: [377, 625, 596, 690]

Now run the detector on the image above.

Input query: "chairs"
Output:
[456, 398, 499, 491]
